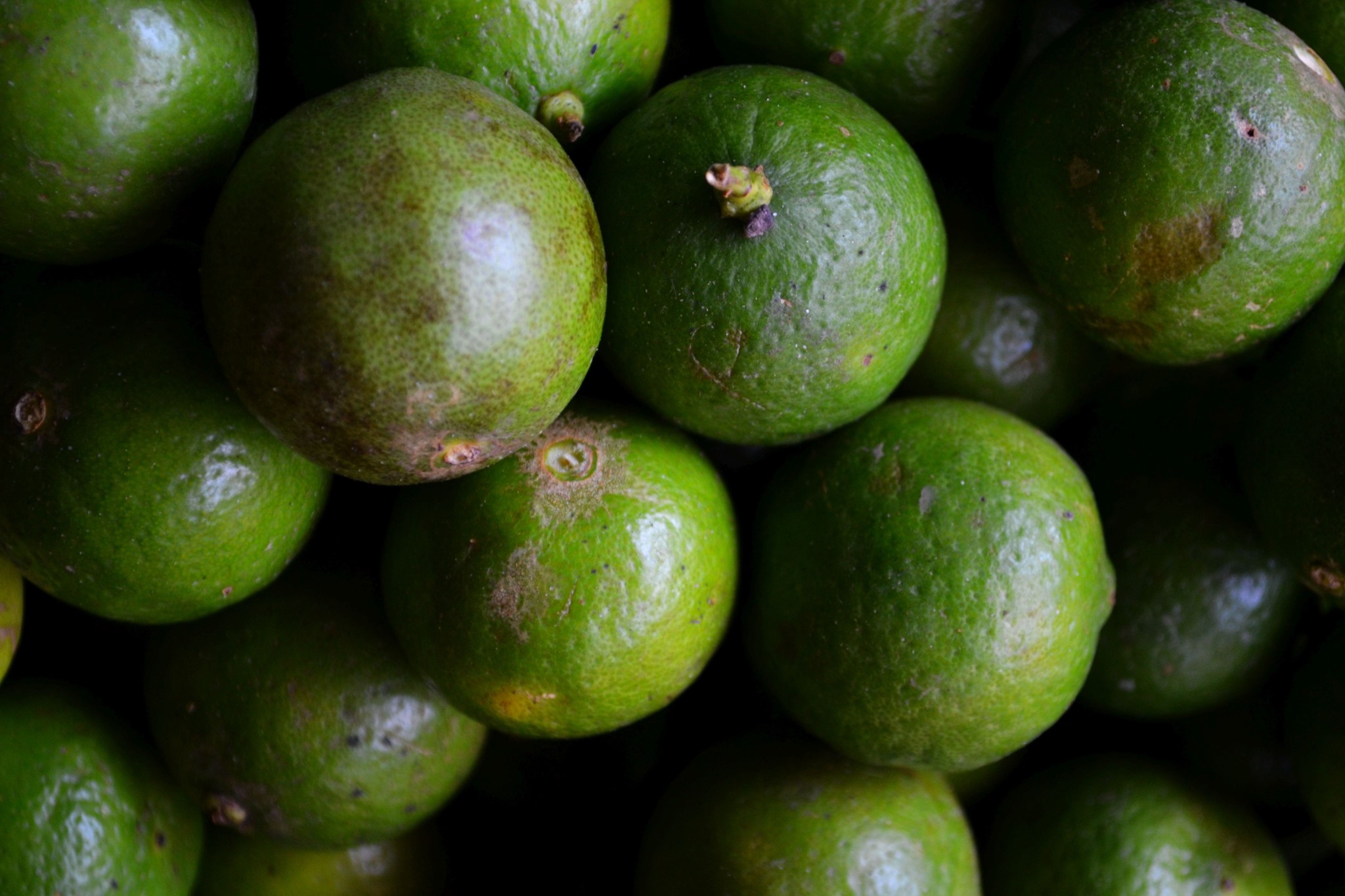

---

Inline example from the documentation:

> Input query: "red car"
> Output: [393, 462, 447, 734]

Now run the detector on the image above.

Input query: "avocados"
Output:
[0, 0, 1345, 896]
